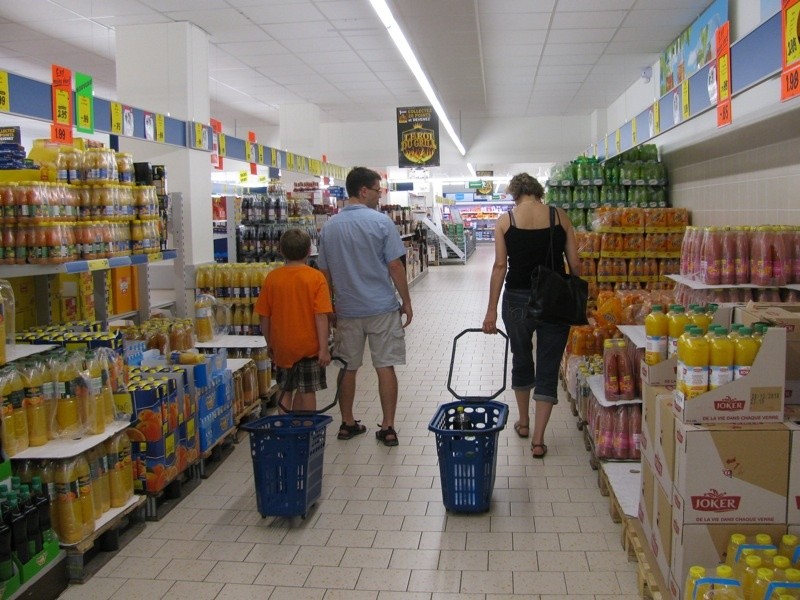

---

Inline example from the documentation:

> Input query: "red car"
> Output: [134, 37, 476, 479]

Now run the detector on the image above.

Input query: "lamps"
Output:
[368, 0, 465, 156]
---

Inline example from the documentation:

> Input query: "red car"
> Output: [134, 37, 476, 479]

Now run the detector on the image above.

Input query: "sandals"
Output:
[375, 424, 398, 446]
[338, 420, 366, 440]
[531, 442, 547, 458]
[514, 421, 528, 438]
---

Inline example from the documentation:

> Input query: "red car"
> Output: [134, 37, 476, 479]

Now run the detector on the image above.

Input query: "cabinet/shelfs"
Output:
[0, 343, 146, 581]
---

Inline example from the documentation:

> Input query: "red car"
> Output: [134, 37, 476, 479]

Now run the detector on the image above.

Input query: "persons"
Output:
[254, 229, 333, 425]
[316, 167, 412, 447]
[481, 172, 581, 459]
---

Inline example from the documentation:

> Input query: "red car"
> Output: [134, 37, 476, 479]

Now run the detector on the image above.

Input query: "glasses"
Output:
[366, 187, 380, 194]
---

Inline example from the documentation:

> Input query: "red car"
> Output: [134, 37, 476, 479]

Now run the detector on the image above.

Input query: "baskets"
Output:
[241, 413, 333, 518]
[428, 399, 509, 510]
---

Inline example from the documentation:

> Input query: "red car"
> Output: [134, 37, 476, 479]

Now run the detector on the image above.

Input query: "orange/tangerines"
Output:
[125, 394, 200, 492]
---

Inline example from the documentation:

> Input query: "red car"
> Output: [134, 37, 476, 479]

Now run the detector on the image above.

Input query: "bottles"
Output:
[0, 140, 338, 592]
[540, 153, 799, 600]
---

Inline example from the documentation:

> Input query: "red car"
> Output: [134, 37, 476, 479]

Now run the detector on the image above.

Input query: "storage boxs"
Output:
[5, 263, 236, 495]
[639, 302, 800, 600]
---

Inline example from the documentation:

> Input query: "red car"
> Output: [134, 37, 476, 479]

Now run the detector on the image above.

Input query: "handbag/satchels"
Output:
[526, 264, 589, 325]
